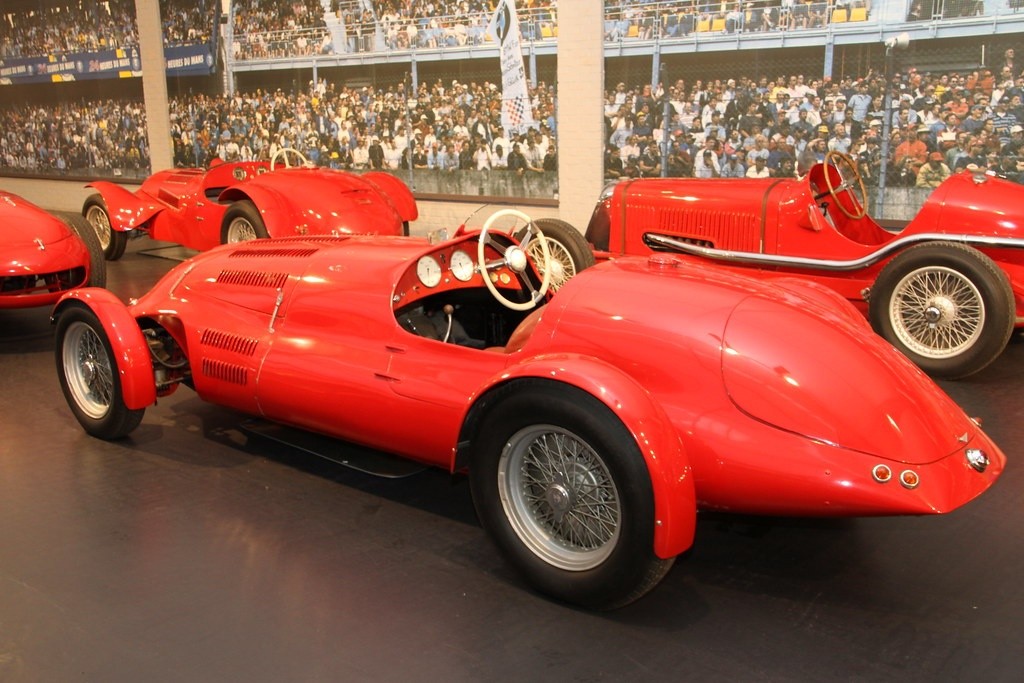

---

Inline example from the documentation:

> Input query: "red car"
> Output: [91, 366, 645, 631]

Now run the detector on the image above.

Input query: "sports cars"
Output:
[0, 185, 107, 308]
[50, 208, 1008, 616]
[83, 149, 421, 263]
[585, 149, 1023, 384]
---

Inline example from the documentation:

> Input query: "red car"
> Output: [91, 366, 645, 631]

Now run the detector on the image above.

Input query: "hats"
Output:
[916, 124, 931, 133]
[817, 125, 829, 132]
[930, 151, 942, 164]
[1010, 125, 1023, 134]
[637, 110, 645, 117]
[868, 119, 883, 128]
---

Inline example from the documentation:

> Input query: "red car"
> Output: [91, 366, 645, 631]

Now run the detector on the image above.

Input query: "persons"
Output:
[603, 0, 1024, 187]
[0, 0, 559, 200]
